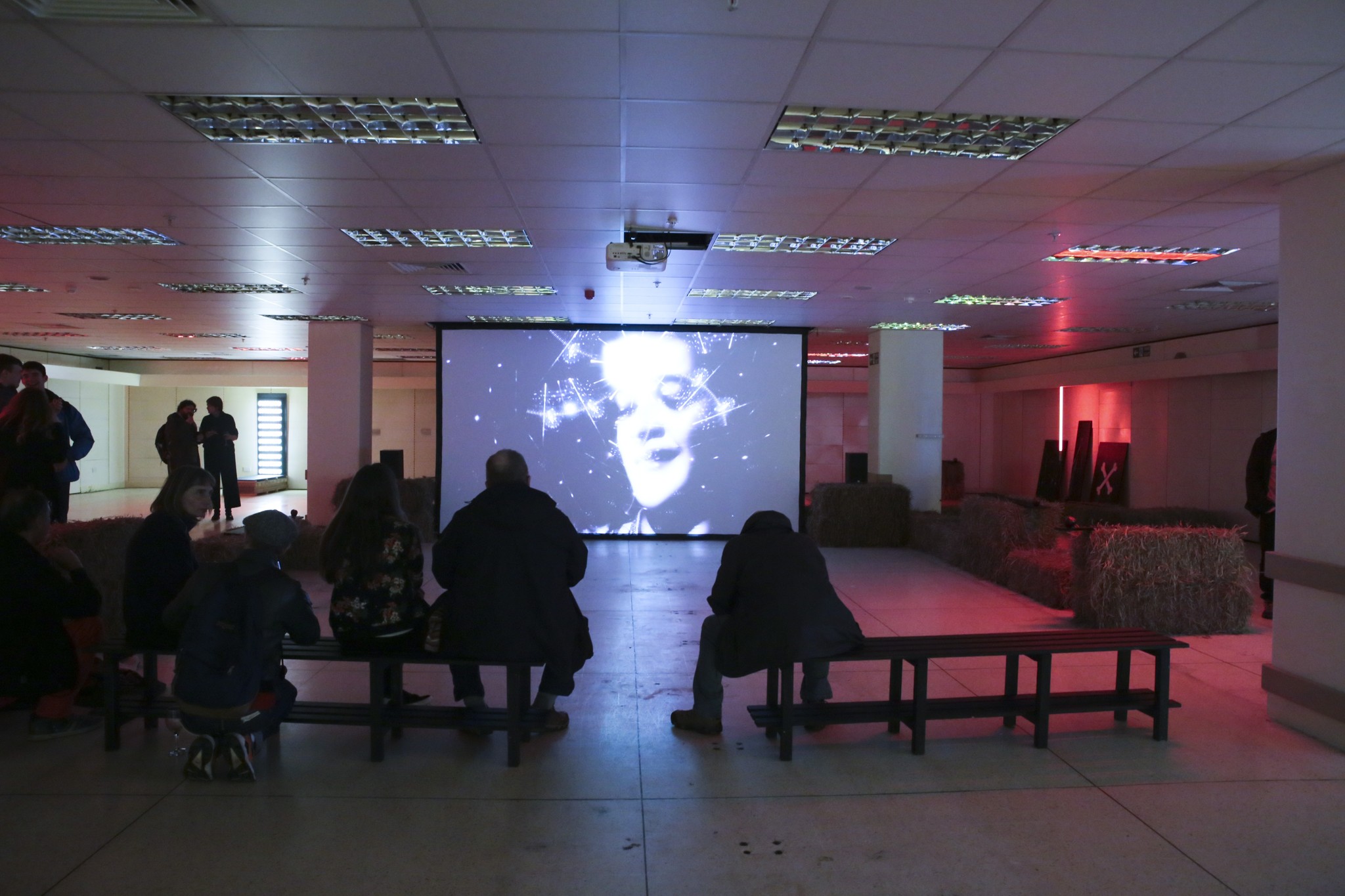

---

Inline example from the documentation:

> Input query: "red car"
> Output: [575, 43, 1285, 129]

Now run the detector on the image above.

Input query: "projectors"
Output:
[605, 242, 668, 272]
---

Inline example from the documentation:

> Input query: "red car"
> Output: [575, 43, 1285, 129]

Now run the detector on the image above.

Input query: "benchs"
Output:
[744, 629, 1189, 758]
[92, 634, 560, 771]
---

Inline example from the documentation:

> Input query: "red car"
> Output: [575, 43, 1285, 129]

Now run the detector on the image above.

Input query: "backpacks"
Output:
[154, 423, 168, 465]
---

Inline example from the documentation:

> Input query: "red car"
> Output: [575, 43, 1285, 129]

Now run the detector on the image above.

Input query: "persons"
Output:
[199, 396, 241, 521]
[0, 352, 24, 414]
[121, 465, 217, 649]
[165, 400, 204, 476]
[1243, 427, 1277, 619]
[430, 449, 596, 738]
[670, 509, 866, 736]
[21, 360, 95, 514]
[163, 509, 320, 782]
[580, 333, 749, 536]
[0, 387, 67, 524]
[322, 463, 435, 707]
[0, 488, 104, 743]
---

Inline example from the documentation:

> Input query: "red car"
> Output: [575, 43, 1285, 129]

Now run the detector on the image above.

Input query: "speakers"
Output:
[845, 452, 868, 484]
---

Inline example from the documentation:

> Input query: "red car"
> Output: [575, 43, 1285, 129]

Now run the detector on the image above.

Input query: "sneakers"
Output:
[27, 713, 97, 740]
[802, 699, 829, 732]
[671, 705, 724, 734]
[184, 734, 217, 782]
[528, 706, 570, 730]
[401, 689, 433, 707]
[222, 733, 257, 782]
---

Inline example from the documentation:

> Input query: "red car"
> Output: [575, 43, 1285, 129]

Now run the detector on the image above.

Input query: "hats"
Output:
[243, 508, 298, 547]
